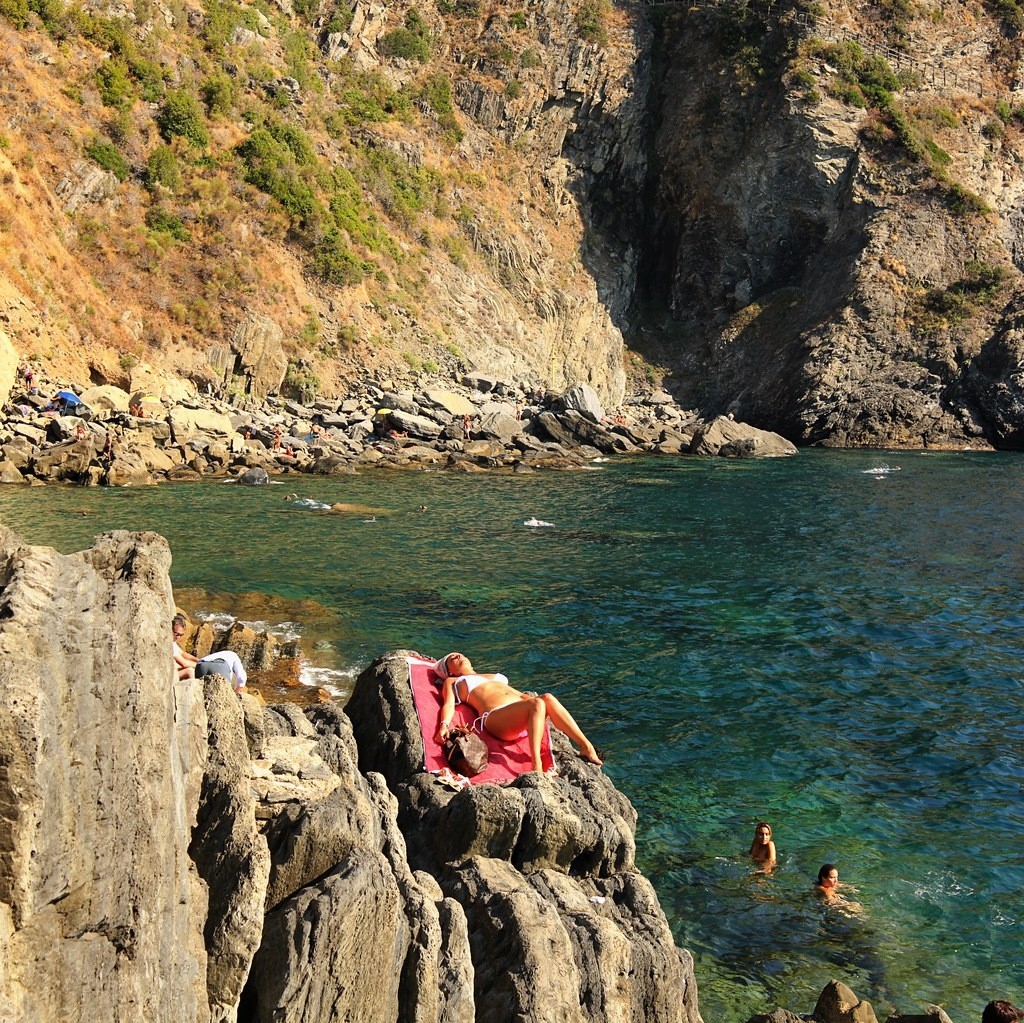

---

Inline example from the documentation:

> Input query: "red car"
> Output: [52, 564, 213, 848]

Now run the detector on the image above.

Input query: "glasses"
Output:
[173, 631, 184, 636]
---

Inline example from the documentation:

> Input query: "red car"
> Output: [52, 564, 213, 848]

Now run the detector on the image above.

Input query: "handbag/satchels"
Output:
[445, 726, 489, 777]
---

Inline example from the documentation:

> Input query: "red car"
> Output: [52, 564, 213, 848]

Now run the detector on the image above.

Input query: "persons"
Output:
[982, 1000, 1024, 1023]
[816, 864, 839, 896]
[105, 433, 111, 461]
[284, 494, 313, 501]
[538, 388, 542, 406]
[287, 443, 294, 456]
[49, 391, 67, 412]
[749, 822, 776, 864]
[729, 412, 734, 420]
[17, 364, 33, 392]
[310, 424, 320, 446]
[172, 613, 248, 695]
[130, 404, 144, 417]
[515, 401, 521, 420]
[434, 652, 603, 775]
[272, 424, 281, 453]
[613, 415, 628, 426]
[464, 416, 472, 440]
[77, 426, 86, 441]
[389, 429, 408, 437]
[244, 428, 251, 438]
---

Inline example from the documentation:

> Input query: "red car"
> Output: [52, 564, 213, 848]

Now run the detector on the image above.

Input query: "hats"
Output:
[433, 651, 466, 678]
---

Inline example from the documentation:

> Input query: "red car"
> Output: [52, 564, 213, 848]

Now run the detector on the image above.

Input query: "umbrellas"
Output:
[58, 392, 80, 415]
